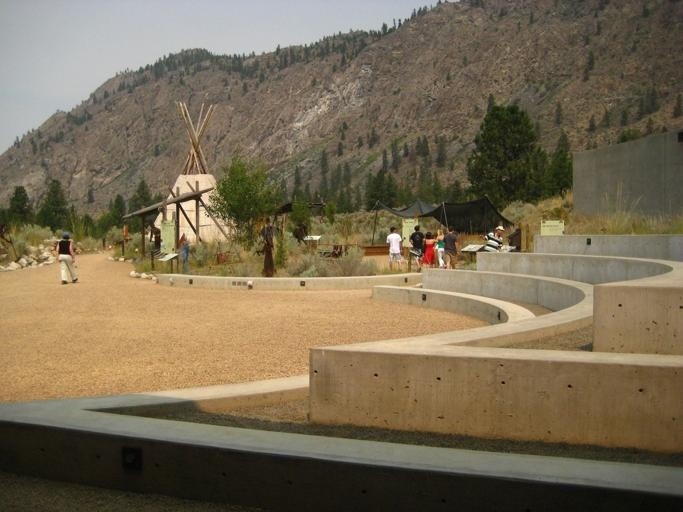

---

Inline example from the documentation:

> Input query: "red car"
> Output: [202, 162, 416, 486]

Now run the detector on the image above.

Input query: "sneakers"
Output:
[62, 278, 78, 285]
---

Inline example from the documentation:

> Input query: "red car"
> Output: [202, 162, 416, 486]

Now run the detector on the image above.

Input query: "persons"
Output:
[422, 232, 437, 268]
[147, 221, 160, 251]
[409, 224, 425, 273]
[384, 227, 404, 274]
[479, 225, 505, 253]
[442, 225, 458, 269]
[54, 231, 78, 284]
[435, 229, 446, 269]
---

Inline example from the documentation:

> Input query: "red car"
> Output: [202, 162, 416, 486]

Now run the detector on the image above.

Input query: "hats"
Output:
[494, 225, 505, 231]
